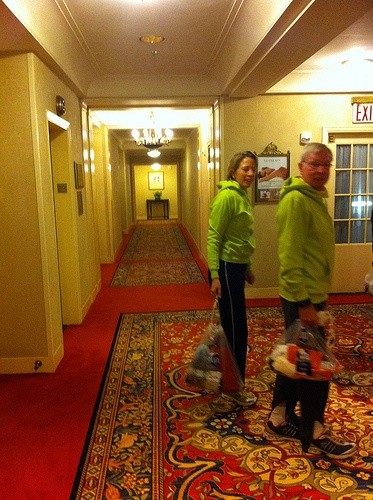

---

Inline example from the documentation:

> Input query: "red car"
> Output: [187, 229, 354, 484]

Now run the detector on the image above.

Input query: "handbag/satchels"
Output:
[264, 311, 345, 381]
[184, 294, 244, 395]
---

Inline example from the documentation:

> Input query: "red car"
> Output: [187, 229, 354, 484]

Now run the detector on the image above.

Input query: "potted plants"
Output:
[153, 190, 162, 200]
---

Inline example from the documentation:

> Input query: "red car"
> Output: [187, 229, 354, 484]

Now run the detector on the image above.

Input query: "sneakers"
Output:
[264, 414, 300, 441]
[221, 388, 257, 406]
[307, 432, 357, 459]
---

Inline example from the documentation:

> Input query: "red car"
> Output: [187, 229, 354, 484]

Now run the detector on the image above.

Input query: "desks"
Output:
[147, 199, 169, 220]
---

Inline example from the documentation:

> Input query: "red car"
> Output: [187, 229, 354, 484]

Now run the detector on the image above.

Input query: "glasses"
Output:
[302, 161, 332, 168]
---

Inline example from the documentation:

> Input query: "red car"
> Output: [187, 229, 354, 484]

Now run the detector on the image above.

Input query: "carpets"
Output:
[69, 301, 373, 500]
[108, 220, 207, 287]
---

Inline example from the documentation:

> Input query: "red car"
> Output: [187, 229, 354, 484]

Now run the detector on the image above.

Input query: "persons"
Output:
[207, 150, 259, 407]
[266, 142, 358, 458]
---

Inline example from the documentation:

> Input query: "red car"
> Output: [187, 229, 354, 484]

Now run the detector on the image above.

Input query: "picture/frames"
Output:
[74, 162, 84, 188]
[147, 171, 165, 190]
[253, 142, 291, 205]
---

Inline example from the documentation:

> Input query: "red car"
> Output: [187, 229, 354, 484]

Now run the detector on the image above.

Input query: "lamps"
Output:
[139, 31, 164, 47]
[131, 110, 174, 158]
